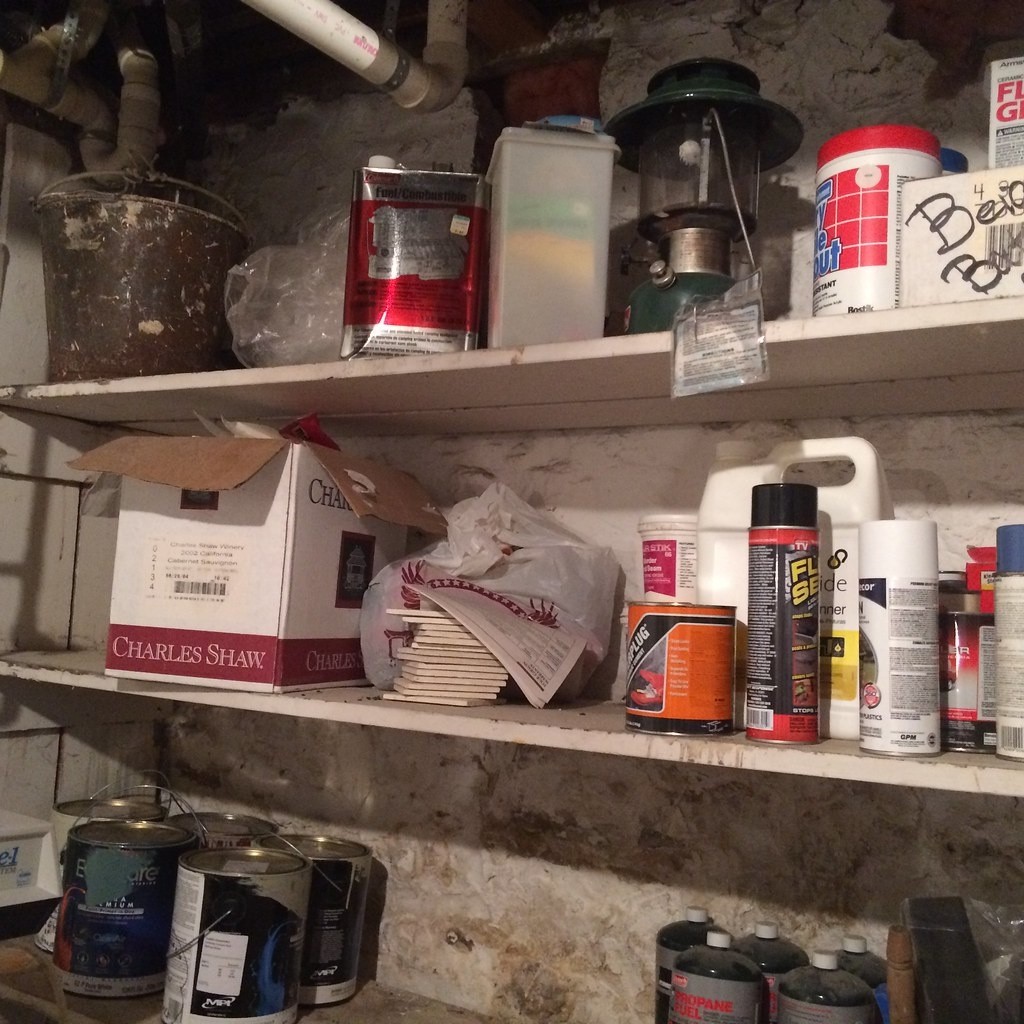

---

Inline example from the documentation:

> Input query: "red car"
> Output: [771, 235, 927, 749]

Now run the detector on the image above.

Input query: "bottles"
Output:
[638, 436, 1024, 763]
[654, 905, 889, 1024]
[812, 123, 942, 316]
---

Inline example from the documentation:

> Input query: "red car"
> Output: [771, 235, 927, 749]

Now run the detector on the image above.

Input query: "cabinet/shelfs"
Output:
[0, 297, 1024, 1024]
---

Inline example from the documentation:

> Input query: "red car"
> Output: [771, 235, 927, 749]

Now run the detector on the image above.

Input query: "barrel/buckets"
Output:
[28, 171, 256, 381]
[696, 436, 895, 741]
[50, 769, 373, 1024]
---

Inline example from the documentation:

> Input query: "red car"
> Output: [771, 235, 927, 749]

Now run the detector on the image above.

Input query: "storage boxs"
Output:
[900, 163, 1024, 307]
[483, 128, 621, 348]
[69, 432, 447, 697]
